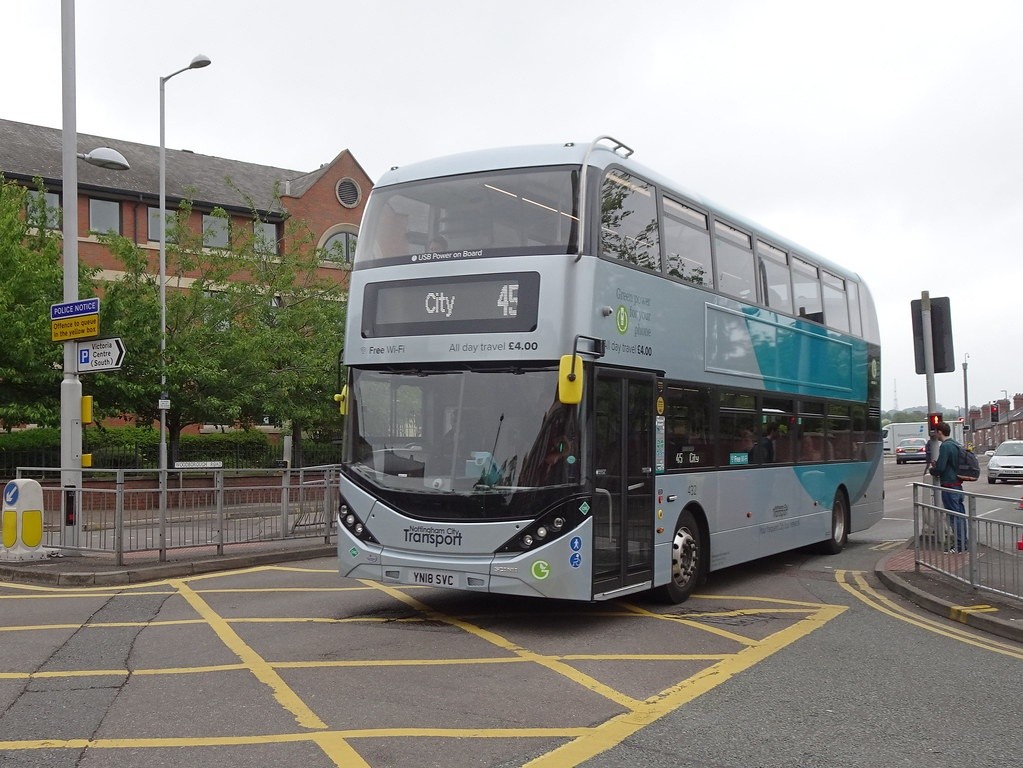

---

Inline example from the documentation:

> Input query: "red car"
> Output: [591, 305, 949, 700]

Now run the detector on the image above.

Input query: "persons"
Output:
[749, 422, 780, 463]
[928, 422, 968, 556]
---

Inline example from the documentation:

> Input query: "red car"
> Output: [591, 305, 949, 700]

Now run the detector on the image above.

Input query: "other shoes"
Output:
[944, 546, 967, 554]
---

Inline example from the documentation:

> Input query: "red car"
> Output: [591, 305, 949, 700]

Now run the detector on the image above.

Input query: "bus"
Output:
[333, 136, 888, 609]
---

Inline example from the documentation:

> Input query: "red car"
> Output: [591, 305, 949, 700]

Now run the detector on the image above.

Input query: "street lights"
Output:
[962, 353, 968, 446]
[158, 53, 212, 563]
[59, 148, 131, 554]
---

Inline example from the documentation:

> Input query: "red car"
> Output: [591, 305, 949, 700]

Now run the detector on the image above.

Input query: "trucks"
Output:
[881, 423, 963, 457]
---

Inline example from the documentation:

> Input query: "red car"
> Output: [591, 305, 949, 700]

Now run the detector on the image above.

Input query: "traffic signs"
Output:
[49, 297, 125, 374]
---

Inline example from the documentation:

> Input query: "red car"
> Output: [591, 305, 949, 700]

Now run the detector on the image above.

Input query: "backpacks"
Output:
[940, 441, 980, 481]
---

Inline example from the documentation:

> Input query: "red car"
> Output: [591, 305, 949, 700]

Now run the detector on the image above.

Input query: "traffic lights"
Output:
[990, 403, 1000, 423]
[963, 425, 970, 431]
[926, 413, 943, 431]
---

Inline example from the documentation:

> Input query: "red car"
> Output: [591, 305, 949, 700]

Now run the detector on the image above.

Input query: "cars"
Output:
[897, 439, 928, 464]
[984, 439, 1023, 483]
